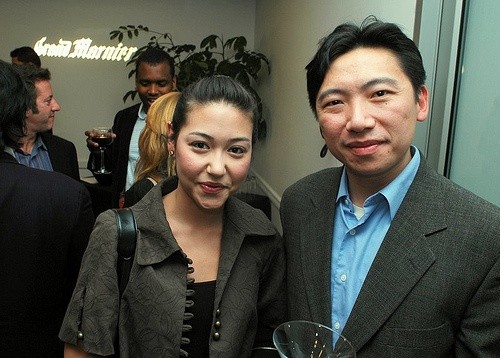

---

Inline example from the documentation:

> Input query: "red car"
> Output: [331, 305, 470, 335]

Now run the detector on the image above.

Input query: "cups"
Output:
[273, 321, 356, 358]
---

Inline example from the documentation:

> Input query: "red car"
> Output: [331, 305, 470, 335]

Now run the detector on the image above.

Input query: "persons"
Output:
[0, 60, 95, 358]
[279, 15, 500, 358]
[5, 47, 80, 180]
[58, 74, 286, 358]
[122, 91, 182, 208]
[85, 47, 176, 209]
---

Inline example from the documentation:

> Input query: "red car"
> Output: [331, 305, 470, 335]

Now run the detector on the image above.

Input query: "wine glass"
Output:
[94, 121, 113, 174]
